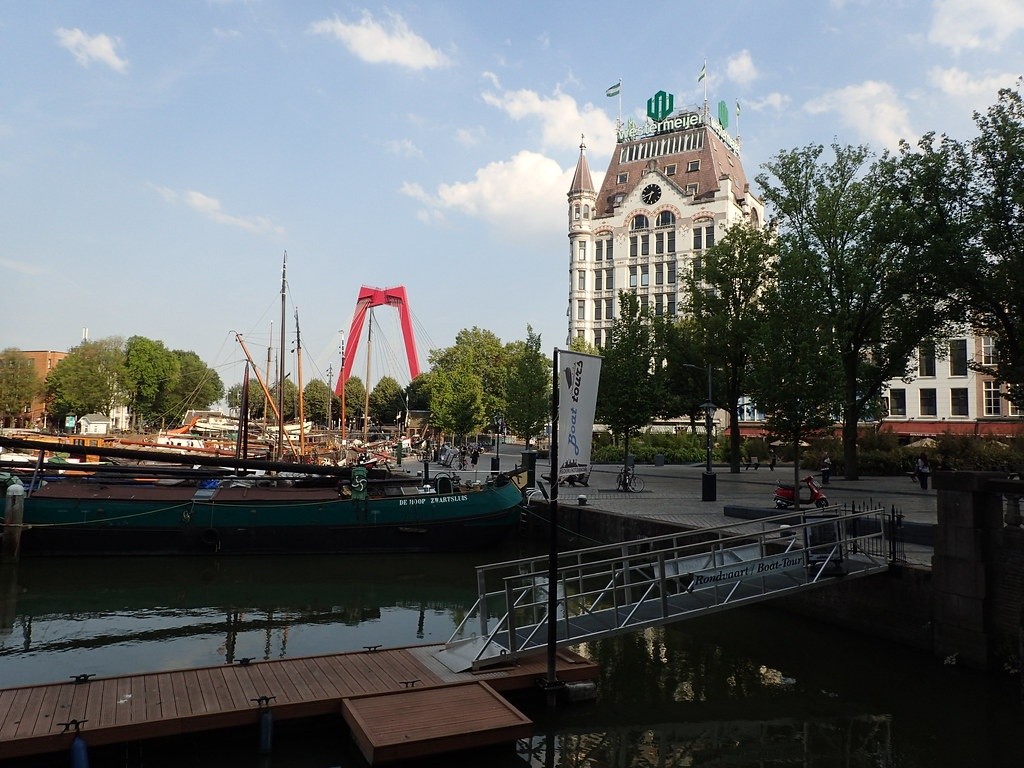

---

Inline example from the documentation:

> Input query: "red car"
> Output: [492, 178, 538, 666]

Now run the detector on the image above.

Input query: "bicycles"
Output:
[453, 456, 473, 471]
[616, 464, 644, 493]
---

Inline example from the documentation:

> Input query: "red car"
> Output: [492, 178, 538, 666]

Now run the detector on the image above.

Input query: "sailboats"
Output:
[0, 249, 527, 555]
[0, 541, 536, 663]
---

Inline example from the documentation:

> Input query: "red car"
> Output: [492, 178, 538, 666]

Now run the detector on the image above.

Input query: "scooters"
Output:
[773, 475, 829, 510]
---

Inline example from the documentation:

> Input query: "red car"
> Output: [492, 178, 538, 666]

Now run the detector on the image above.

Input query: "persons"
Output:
[470, 446, 479, 468]
[558, 473, 585, 487]
[821, 451, 833, 485]
[768, 454, 777, 471]
[911, 452, 929, 490]
[459, 442, 466, 471]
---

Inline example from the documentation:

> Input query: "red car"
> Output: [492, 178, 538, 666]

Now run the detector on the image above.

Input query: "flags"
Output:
[736, 102, 743, 116]
[605, 82, 620, 98]
[697, 64, 706, 83]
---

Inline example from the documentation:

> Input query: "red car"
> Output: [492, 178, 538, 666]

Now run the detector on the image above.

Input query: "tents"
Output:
[769, 437, 812, 447]
[903, 437, 940, 449]
[984, 440, 1009, 450]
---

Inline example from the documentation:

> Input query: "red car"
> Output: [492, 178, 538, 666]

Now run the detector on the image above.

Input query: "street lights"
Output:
[683, 363, 718, 501]
[491, 415, 503, 475]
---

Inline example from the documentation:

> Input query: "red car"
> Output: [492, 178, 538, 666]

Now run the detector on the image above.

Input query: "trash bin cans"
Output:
[655, 454, 664, 466]
[628, 455, 633, 465]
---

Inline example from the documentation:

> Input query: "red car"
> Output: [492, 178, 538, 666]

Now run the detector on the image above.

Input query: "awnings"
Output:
[878, 422, 1024, 439]
[721, 426, 869, 441]
